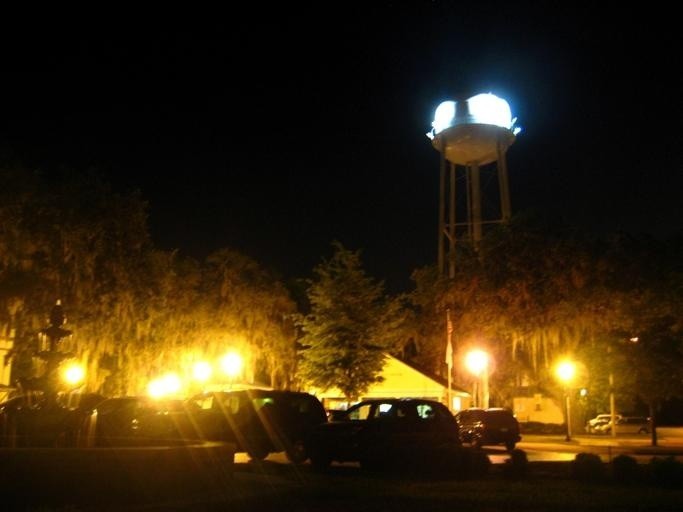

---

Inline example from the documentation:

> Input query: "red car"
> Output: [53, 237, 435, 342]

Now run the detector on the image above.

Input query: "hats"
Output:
[303, 398, 466, 473]
[452, 407, 522, 452]
[586, 413, 625, 426]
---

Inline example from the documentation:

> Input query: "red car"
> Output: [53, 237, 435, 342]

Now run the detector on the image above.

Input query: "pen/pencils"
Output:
[594, 416, 654, 437]
